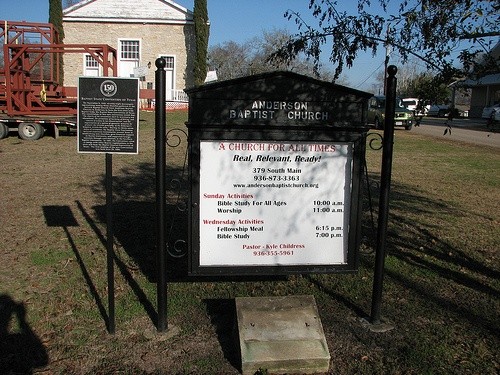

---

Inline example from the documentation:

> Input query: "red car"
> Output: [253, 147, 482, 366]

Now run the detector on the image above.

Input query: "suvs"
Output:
[368, 95, 414, 130]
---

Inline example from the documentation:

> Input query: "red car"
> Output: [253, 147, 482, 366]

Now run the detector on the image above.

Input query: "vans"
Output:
[399, 98, 452, 117]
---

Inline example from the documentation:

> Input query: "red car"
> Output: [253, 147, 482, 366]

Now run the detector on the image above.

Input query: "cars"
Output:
[481, 103, 500, 123]
